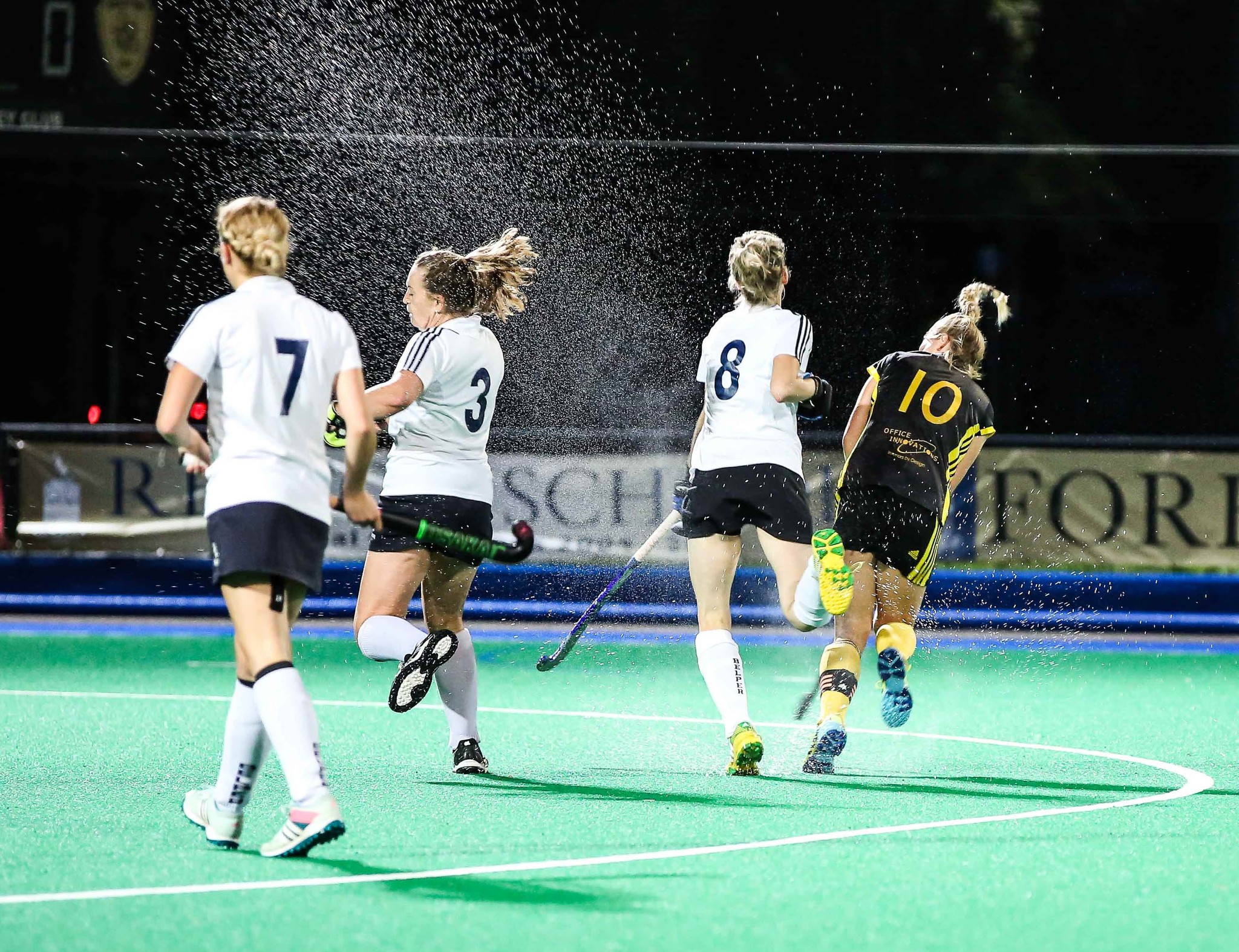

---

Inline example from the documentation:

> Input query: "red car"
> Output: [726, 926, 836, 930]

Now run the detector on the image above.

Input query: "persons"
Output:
[669, 229, 855, 777]
[323, 226, 540, 776]
[155, 194, 382, 857]
[801, 282, 1011, 772]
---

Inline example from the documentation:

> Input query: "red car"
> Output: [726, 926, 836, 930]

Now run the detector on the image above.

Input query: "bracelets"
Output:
[810, 377, 817, 396]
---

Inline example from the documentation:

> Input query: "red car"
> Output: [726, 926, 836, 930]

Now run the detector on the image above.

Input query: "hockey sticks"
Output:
[177, 451, 534, 565]
[535, 495, 688, 672]
[793, 681, 820, 720]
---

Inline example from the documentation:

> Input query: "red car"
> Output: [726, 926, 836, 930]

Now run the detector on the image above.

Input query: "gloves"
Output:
[672, 483, 692, 537]
[799, 373, 833, 422]
[324, 402, 354, 450]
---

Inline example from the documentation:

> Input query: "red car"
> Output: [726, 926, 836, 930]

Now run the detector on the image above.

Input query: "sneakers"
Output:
[260, 792, 345, 857]
[182, 788, 244, 849]
[452, 739, 490, 776]
[802, 719, 846, 774]
[876, 646, 912, 729]
[389, 626, 459, 713]
[726, 727, 765, 776]
[811, 528, 853, 618]
